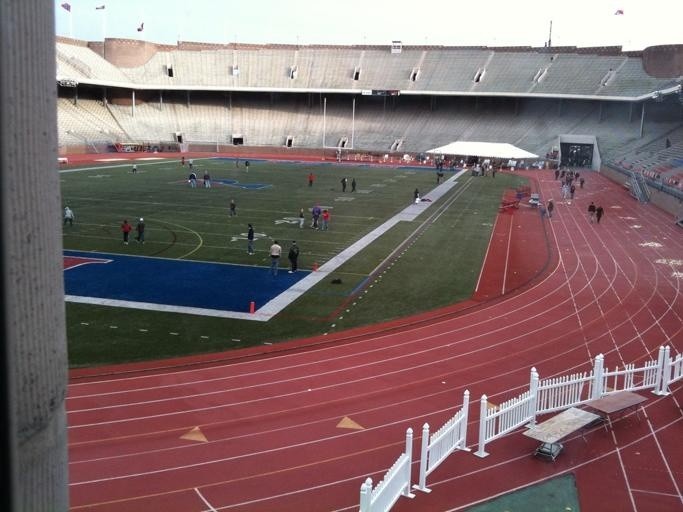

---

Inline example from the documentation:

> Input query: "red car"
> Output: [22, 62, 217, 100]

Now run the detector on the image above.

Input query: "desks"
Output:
[521, 407, 601, 462]
[585, 391, 649, 432]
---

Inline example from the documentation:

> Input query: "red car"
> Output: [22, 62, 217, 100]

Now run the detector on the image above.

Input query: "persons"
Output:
[268, 240, 282, 276]
[133, 163, 137, 175]
[309, 172, 315, 187]
[229, 198, 237, 219]
[181, 154, 210, 188]
[435, 159, 497, 184]
[287, 241, 300, 273]
[537, 165, 604, 224]
[64, 206, 75, 226]
[340, 176, 347, 191]
[245, 160, 250, 172]
[351, 177, 356, 193]
[120, 220, 131, 244]
[246, 223, 255, 256]
[414, 188, 420, 204]
[298, 202, 331, 230]
[135, 217, 146, 245]
